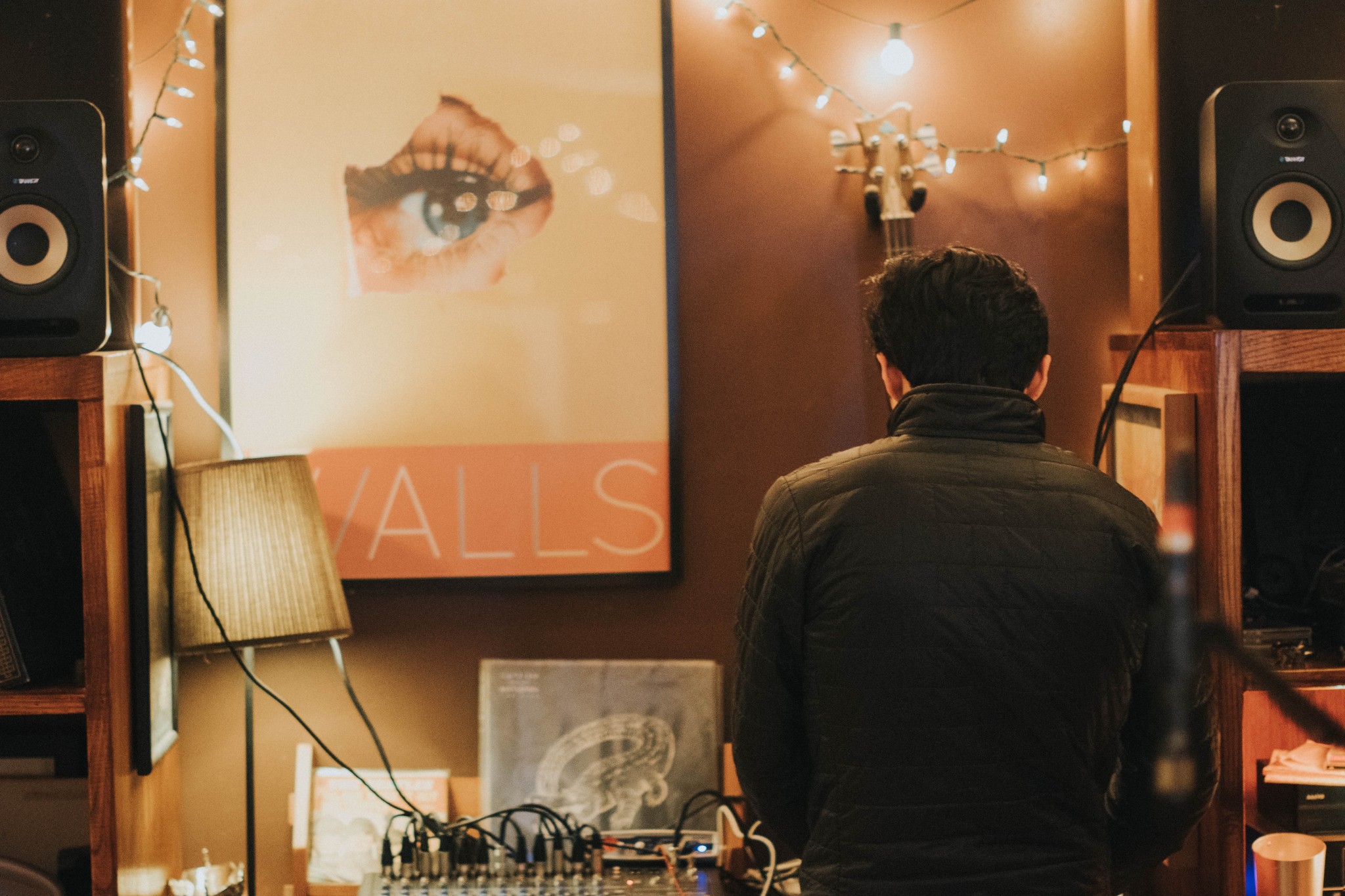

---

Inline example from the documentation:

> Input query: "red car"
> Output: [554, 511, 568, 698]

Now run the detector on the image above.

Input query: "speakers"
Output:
[1197, 79, 1345, 329]
[0, 100, 113, 359]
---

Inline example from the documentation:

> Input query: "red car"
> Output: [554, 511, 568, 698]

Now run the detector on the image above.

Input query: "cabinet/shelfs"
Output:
[0, 348, 186, 896]
[1113, 329, 1345, 896]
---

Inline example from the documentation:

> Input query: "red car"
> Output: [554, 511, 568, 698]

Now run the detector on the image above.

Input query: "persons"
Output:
[729, 243, 1162, 896]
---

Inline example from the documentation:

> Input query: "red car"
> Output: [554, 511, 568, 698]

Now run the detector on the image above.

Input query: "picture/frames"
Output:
[1099, 382, 1199, 551]
[127, 398, 179, 775]
[210, 0, 687, 588]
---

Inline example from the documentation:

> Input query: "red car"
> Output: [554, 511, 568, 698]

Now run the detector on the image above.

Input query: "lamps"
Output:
[175, 454, 354, 896]
